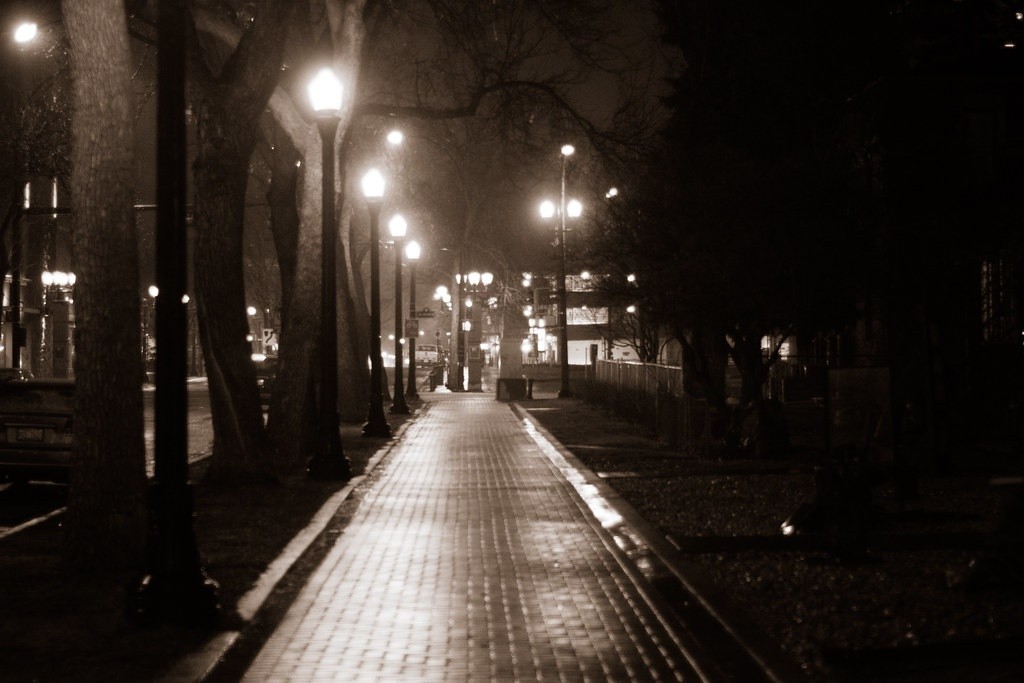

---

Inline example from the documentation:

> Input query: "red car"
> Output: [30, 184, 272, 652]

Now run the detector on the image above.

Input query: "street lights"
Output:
[304, 66, 348, 484]
[359, 164, 393, 439]
[387, 212, 413, 415]
[404, 238, 422, 403]
[538, 143, 584, 399]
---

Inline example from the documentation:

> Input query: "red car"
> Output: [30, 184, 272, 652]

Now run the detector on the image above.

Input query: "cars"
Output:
[415, 344, 445, 368]
[0, 367, 78, 483]
[255, 355, 279, 397]
